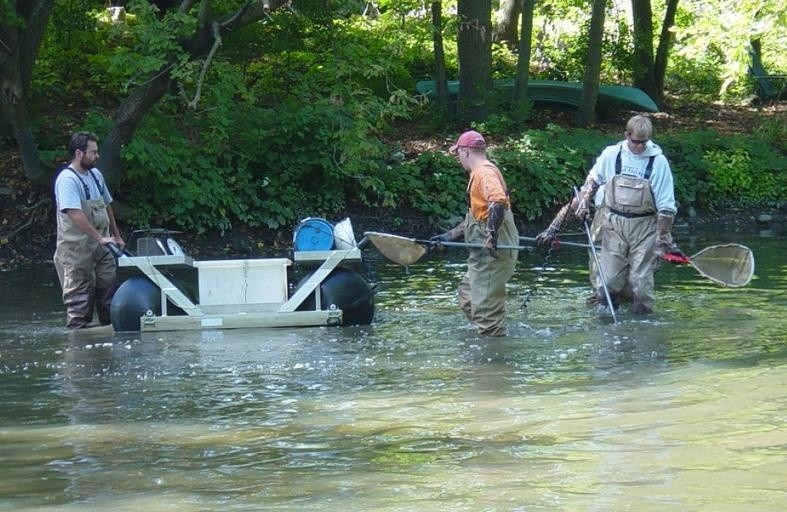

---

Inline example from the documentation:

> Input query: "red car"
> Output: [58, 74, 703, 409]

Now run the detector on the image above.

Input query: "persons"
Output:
[573, 115, 677, 319]
[53, 131, 126, 330]
[431, 131, 521, 340]
[537, 177, 684, 307]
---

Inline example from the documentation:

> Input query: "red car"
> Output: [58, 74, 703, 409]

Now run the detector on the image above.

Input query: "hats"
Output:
[450, 130, 487, 151]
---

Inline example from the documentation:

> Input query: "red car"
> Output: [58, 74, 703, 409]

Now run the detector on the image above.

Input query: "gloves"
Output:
[534, 203, 575, 256]
[667, 243, 687, 268]
[427, 219, 466, 250]
[656, 211, 674, 266]
[482, 199, 506, 260]
[574, 175, 598, 223]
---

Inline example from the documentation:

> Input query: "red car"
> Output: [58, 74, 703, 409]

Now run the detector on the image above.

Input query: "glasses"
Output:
[629, 135, 649, 144]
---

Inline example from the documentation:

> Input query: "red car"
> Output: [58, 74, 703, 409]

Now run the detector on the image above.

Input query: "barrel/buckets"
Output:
[293, 217, 334, 251]
